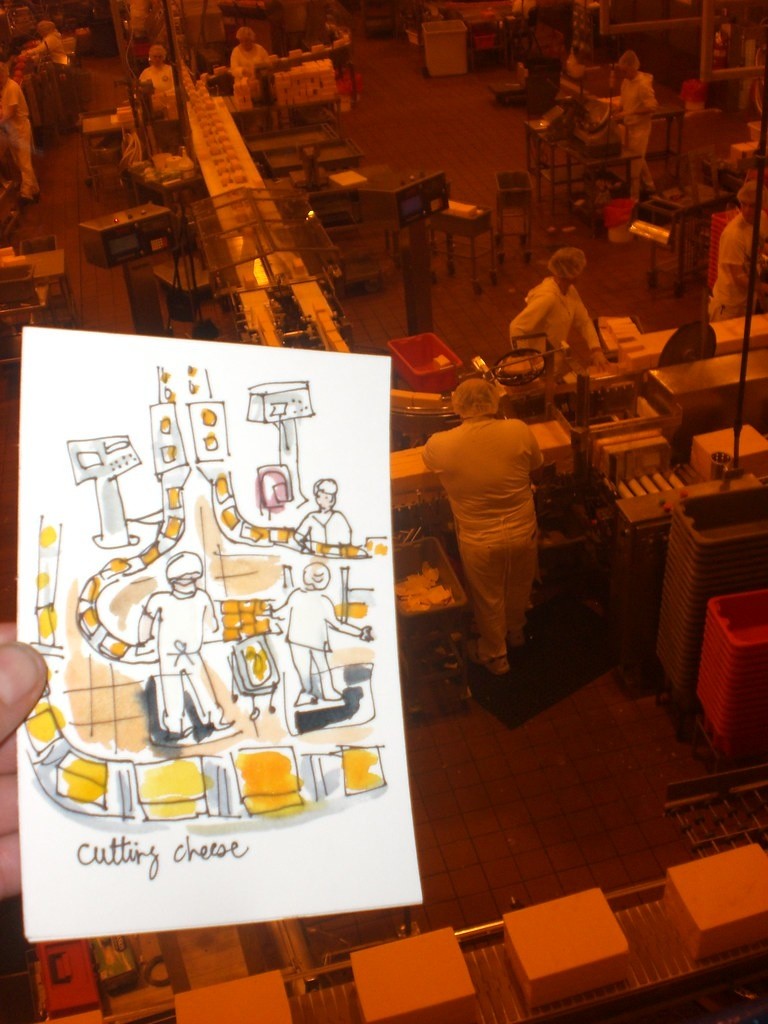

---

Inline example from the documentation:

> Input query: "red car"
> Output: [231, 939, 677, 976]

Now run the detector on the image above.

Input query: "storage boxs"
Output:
[708, 209, 740, 292]
[422, 20, 468, 75]
[387, 331, 463, 393]
[393, 537, 468, 648]
[657, 485, 768, 760]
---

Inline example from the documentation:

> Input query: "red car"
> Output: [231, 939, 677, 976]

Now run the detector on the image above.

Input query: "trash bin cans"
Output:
[18, 235, 58, 255]
[420, 19, 469, 77]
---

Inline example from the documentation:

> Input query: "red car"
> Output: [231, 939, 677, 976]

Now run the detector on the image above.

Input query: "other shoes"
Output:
[32, 192, 41, 203]
[18, 197, 33, 205]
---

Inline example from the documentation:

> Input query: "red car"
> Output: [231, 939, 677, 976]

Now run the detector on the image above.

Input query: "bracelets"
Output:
[590, 347, 602, 354]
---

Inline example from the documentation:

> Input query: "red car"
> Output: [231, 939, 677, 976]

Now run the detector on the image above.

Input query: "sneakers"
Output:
[500, 625, 524, 648]
[464, 638, 511, 676]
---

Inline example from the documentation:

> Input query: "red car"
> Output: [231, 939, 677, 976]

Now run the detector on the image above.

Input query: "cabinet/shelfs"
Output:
[82, 115, 206, 253]
[0, 249, 79, 369]
[430, 92, 687, 292]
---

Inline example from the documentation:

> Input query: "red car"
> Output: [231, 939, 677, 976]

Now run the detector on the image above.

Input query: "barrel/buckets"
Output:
[152, 153, 171, 170]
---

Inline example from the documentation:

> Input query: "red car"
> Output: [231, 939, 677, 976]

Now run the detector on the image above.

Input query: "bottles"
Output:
[179, 147, 194, 178]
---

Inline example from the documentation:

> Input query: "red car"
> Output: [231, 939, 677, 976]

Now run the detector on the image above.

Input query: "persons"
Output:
[25, 20, 75, 108]
[708, 179, 768, 322]
[510, 247, 611, 373]
[421, 378, 546, 675]
[138, 45, 174, 92]
[0, 63, 41, 205]
[610, 50, 657, 203]
[230, 27, 269, 72]
